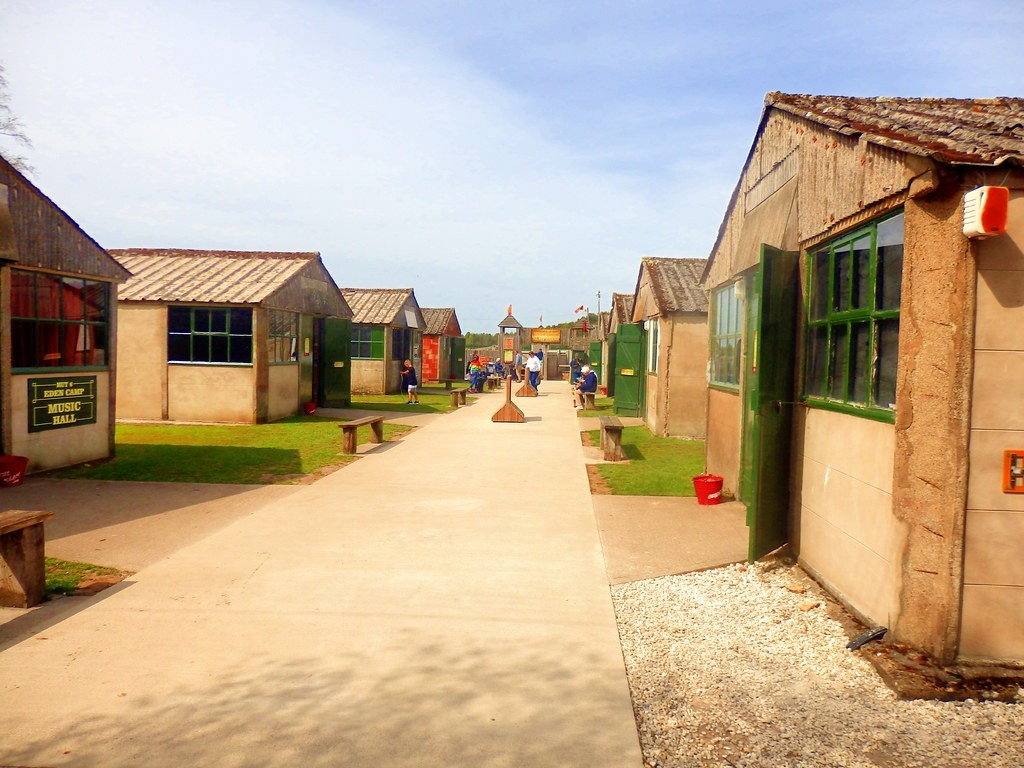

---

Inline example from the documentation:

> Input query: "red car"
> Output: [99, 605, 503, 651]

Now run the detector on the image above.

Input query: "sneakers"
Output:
[574, 405, 583, 409]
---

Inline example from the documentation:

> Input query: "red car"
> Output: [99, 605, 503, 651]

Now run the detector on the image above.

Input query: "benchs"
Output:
[338, 416, 385, 455]
[0, 510, 54, 610]
[488, 377, 502, 390]
[428, 378, 464, 389]
[449, 387, 468, 408]
[598, 416, 624, 461]
[581, 392, 596, 410]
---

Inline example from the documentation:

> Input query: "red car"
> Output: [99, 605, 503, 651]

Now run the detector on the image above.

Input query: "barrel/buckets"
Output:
[0, 456, 29, 487]
[600, 387, 606, 395]
[692, 474, 723, 505]
[304, 402, 316, 415]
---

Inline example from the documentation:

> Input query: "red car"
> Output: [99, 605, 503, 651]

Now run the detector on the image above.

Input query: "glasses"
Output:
[529, 353, 532, 355]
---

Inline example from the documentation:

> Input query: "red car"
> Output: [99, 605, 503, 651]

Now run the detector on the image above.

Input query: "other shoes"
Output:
[475, 388, 481, 392]
[468, 388, 472, 393]
[413, 401, 419, 404]
[517, 380, 522, 383]
[406, 401, 412, 404]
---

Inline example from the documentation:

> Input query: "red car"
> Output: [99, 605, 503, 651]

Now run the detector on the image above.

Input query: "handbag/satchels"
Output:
[534, 377, 540, 385]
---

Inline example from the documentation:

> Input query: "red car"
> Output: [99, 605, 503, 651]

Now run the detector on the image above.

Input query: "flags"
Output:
[575, 305, 584, 313]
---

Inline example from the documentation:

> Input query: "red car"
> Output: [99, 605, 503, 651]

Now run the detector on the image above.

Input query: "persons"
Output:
[400, 359, 419, 405]
[570, 353, 598, 410]
[466, 347, 543, 395]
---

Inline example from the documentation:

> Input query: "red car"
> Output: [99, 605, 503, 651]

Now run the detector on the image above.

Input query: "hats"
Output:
[580, 366, 589, 372]
[496, 358, 500, 362]
